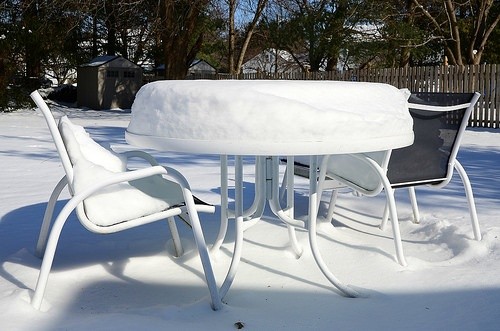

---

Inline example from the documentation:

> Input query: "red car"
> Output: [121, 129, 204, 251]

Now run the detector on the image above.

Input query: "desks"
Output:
[125, 127, 415, 301]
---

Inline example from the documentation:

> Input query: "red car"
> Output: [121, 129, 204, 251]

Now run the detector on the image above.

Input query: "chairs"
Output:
[309, 92, 483, 266]
[277, 156, 363, 225]
[28, 90, 225, 312]
